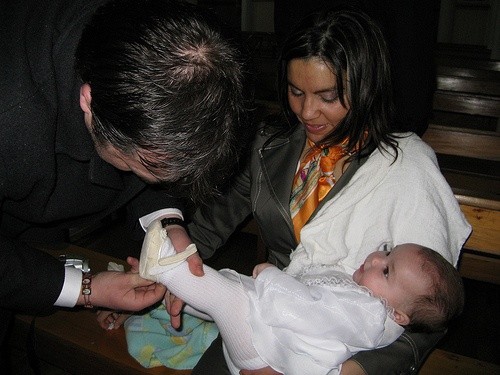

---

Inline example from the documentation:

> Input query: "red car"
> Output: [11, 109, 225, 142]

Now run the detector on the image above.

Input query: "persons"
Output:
[0, 0, 240, 311]
[96, 6, 474, 375]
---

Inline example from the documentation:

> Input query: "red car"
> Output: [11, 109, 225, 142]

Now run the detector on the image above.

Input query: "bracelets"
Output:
[81, 272, 94, 309]
[160, 217, 191, 236]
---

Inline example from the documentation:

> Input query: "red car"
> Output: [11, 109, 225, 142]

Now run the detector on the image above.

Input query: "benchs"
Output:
[12, 60, 500, 375]
